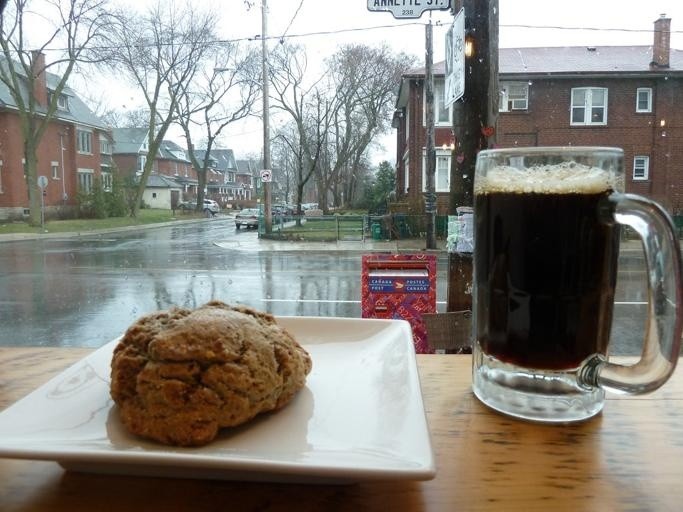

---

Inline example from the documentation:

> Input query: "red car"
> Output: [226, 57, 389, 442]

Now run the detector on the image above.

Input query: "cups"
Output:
[468, 144, 683, 426]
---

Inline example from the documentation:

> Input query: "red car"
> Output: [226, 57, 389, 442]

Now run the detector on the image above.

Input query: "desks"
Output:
[0, 345, 683, 512]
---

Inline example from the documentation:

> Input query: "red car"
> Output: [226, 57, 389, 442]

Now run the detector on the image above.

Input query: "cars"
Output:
[233, 203, 318, 231]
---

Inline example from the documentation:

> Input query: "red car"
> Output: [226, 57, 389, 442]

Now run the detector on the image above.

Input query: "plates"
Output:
[1, 311, 438, 486]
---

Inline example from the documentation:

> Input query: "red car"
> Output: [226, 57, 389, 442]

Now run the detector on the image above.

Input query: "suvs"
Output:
[176, 199, 219, 217]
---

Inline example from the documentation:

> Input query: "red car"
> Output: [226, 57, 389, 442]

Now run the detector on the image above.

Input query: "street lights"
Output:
[213, 65, 272, 235]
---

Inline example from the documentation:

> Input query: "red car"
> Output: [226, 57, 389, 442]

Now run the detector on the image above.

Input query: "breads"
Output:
[109, 299, 313, 445]
